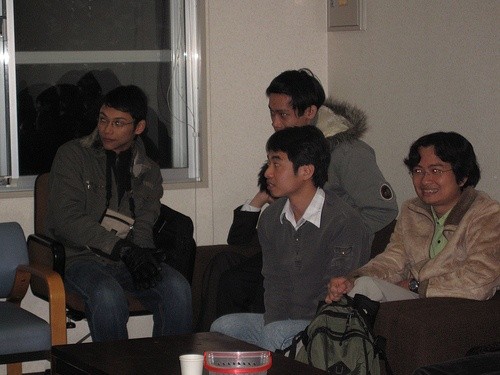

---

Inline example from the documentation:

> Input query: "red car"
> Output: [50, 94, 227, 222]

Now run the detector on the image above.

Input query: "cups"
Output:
[179, 354, 204, 375]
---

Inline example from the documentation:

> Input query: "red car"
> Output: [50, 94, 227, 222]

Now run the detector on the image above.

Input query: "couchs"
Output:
[195, 219, 500, 375]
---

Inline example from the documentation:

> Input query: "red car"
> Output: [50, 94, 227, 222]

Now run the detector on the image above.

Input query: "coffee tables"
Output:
[50, 331, 332, 375]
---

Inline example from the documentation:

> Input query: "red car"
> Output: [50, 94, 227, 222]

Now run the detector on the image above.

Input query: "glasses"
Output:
[408, 167, 455, 178]
[96, 115, 137, 128]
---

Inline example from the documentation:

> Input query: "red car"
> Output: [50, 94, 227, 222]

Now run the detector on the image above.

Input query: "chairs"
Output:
[28, 171, 197, 343]
[0, 221, 69, 375]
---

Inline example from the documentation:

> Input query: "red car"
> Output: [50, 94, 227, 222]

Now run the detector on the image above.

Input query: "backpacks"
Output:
[279, 293, 391, 375]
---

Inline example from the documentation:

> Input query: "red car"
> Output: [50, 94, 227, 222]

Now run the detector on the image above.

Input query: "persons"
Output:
[208, 125, 370, 352]
[44, 85, 193, 342]
[283, 132, 500, 359]
[266, 68, 398, 247]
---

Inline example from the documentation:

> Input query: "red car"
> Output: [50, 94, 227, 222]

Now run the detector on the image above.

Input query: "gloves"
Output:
[122, 247, 164, 290]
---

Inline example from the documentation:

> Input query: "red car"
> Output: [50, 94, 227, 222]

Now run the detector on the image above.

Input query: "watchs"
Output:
[408, 278, 419, 293]
